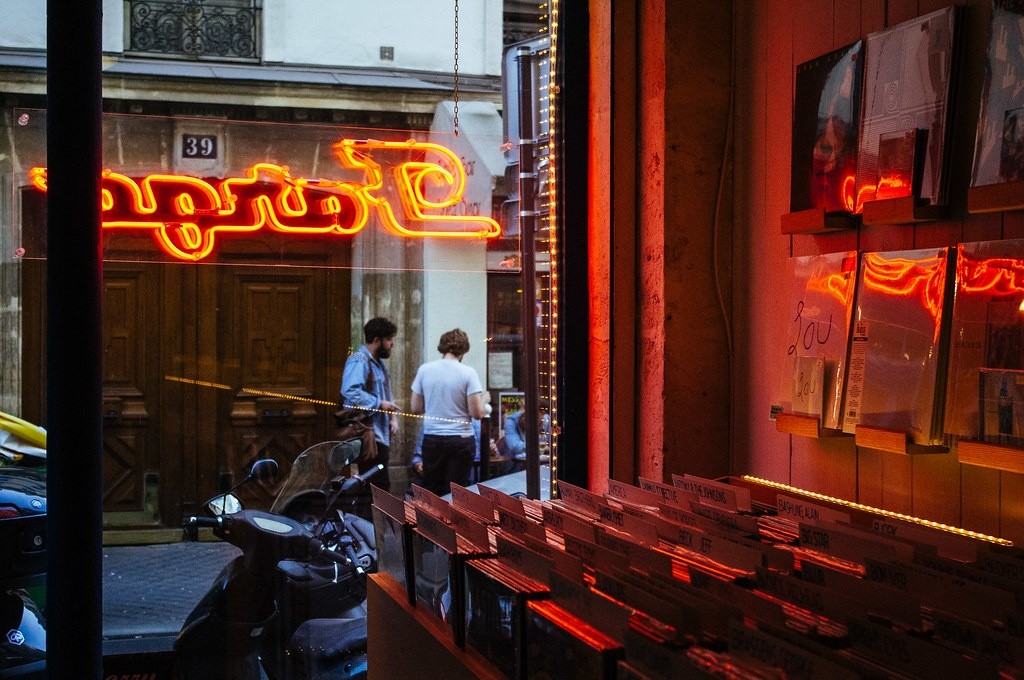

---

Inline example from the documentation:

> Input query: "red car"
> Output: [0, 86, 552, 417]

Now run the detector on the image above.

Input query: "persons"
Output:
[339, 316, 402, 519]
[997, 374, 1013, 447]
[411, 413, 483, 488]
[502, 398, 552, 475]
[410, 328, 490, 496]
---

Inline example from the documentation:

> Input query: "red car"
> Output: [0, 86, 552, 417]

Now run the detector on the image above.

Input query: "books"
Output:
[370, 473, 1024, 680]
[770, 2, 1023, 449]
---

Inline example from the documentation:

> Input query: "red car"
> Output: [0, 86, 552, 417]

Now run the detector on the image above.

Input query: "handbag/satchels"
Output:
[334, 410, 379, 463]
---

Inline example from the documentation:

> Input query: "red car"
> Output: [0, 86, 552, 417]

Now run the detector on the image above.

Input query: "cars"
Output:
[0, 413, 47, 666]
[439, 461, 553, 506]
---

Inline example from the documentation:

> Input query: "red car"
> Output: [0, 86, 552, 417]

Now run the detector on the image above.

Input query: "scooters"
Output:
[172, 436, 386, 680]
